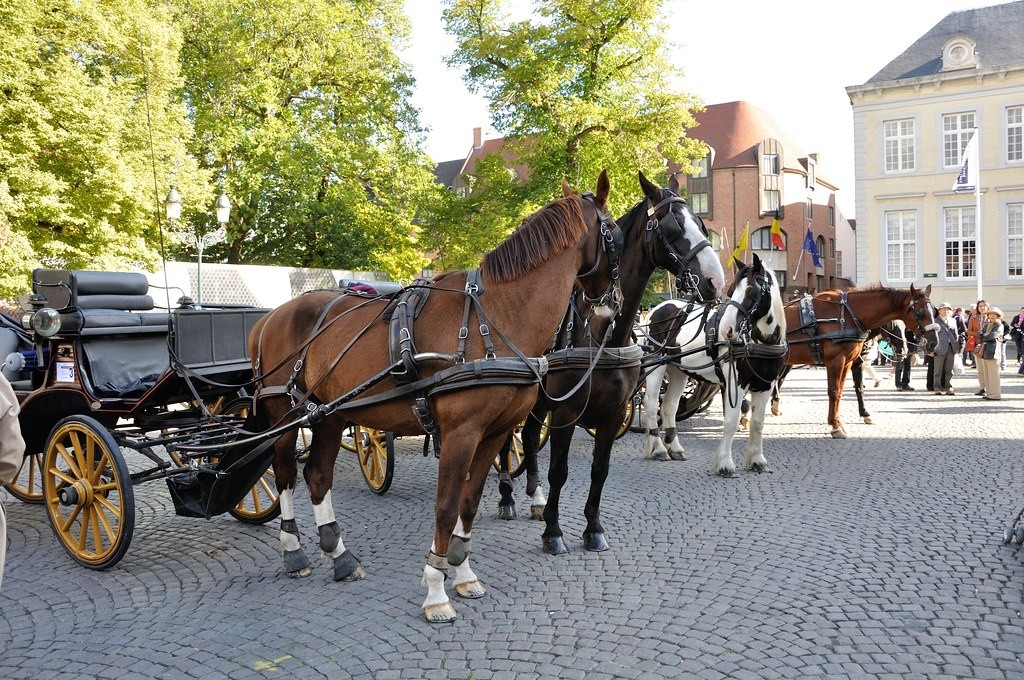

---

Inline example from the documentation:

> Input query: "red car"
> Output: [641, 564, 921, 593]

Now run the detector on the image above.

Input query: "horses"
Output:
[739, 283, 940, 439]
[499, 169, 726, 554]
[248, 166, 625, 624]
[641, 252, 787, 479]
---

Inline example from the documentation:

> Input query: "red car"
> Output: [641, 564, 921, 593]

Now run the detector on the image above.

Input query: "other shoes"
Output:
[874, 379, 883, 388]
[975, 389, 985, 395]
[935, 391, 942, 395]
[897, 385, 903, 391]
[852, 384, 866, 388]
[981, 396, 1002, 400]
[970, 363, 976, 369]
[871, 362, 878, 365]
[946, 387, 954, 395]
[904, 385, 915, 391]
[880, 363, 886, 365]
[927, 388, 934, 391]
[963, 362, 970, 366]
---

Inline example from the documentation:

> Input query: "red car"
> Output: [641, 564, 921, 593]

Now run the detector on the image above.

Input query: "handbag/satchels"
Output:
[1011, 332, 1020, 343]
[966, 316, 977, 350]
[974, 342, 986, 358]
[950, 353, 963, 375]
[1004, 334, 1012, 341]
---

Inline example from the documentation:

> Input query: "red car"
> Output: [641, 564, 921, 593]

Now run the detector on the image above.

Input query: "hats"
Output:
[937, 302, 952, 310]
[987, 307, 1002, 317]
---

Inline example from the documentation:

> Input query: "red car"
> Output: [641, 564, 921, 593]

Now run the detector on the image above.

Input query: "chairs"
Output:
[0, 327, 56, 406]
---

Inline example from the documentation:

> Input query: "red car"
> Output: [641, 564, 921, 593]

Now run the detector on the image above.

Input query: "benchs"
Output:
[33, 269, 168, 339]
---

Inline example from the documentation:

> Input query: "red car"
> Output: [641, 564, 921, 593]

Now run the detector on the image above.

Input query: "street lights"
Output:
[166, 184, 230, 300]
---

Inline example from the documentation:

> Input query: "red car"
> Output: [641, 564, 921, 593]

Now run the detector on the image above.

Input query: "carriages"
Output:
[1, 171, 940, 625]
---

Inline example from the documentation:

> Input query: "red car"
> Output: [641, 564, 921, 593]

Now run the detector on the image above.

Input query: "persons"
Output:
[869, 299, 1024, 401]
[0, 368, 25, 598]
